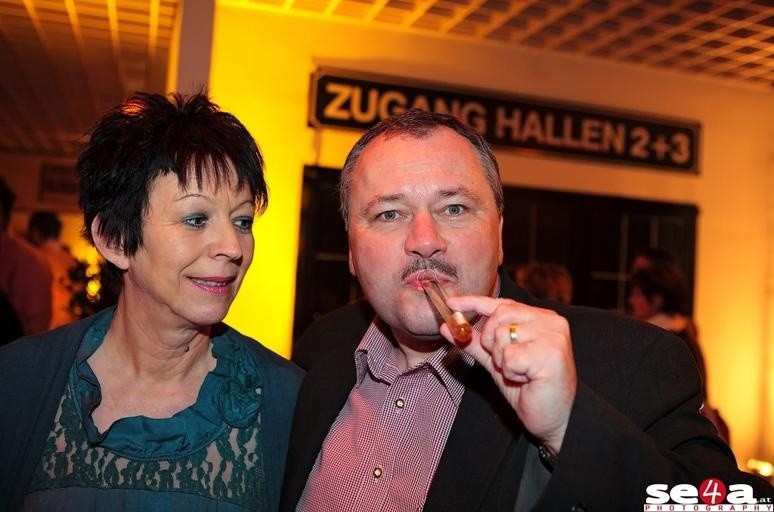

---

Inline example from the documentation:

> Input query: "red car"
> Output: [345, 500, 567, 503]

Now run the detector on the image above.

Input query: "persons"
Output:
[0, 178, 87, 346]
[276, 109, 744, 512]
[628, 243, 707, 413]
[516, 259, 575, 307]
[0, 90, 308, 512]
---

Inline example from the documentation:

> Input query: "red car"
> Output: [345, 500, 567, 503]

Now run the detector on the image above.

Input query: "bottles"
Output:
[416, 269, 473, 349]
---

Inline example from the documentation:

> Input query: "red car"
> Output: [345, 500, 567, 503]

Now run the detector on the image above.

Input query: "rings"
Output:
[509, 322, 519, 342]
